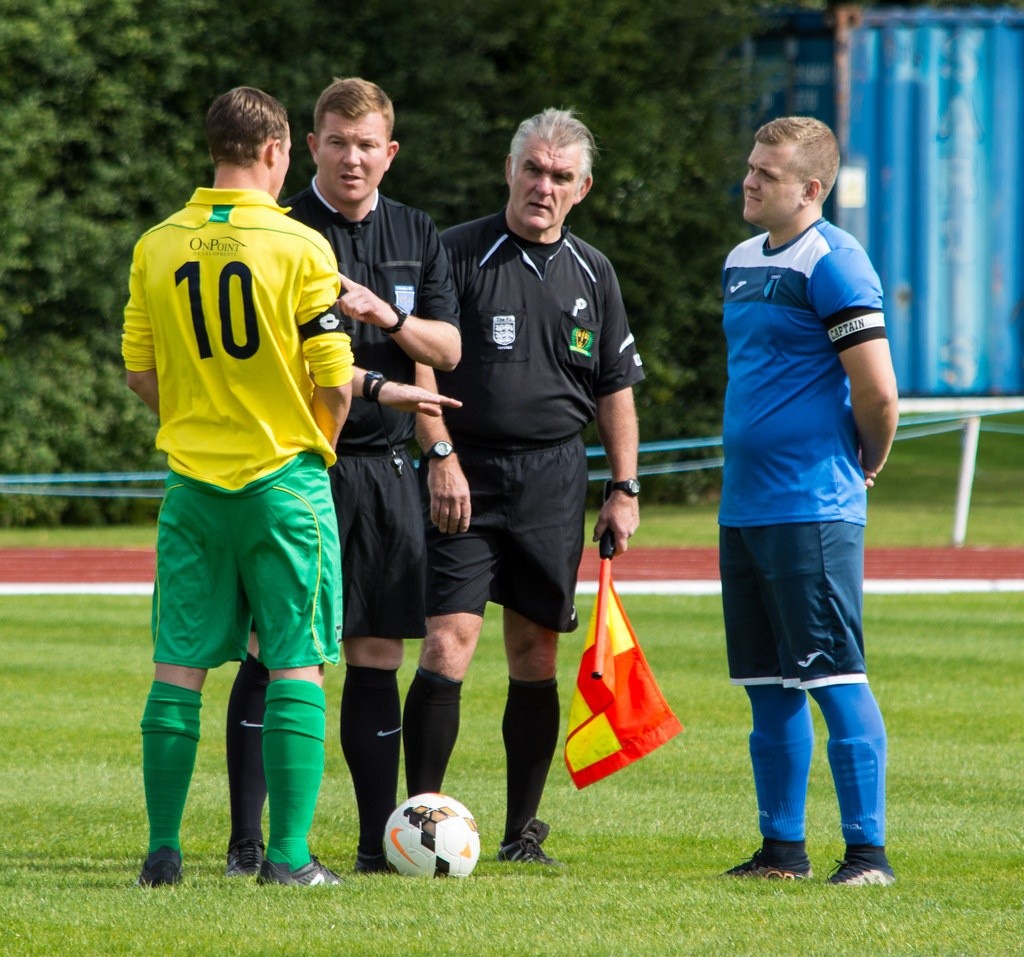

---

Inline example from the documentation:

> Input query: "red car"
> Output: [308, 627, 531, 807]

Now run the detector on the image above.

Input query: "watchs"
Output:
[426, 441, 455, 461]
[611, 479, 641, 497]
[380, 303, 408, 333]
[360, 370, 383, 401]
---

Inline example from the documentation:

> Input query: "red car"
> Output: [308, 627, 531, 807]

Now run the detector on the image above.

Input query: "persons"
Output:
[718, 117, 899, 883]
[225, 78, 462, 878]
[400, 107, 646, 867]
[122, 85, 353, 889]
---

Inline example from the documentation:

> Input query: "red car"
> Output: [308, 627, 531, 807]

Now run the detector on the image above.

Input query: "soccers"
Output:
[382, 792, 481, 879]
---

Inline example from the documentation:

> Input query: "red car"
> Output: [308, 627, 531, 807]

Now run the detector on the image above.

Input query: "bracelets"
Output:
[371, 379, 388, 402]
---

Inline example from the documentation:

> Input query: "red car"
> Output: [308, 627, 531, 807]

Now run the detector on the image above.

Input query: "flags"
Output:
[564, 575, 683, 791]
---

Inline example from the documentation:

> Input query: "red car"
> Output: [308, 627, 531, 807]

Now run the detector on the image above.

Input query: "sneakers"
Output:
[226, 838, 265, 878]
[497, 817, 563, 866]
[825, 859, 896, 886]
[139, 846, 182, 890]
[354, 846, 389, 873]
[725, 848, 813, 881]
[257, 855, 349, 890]
